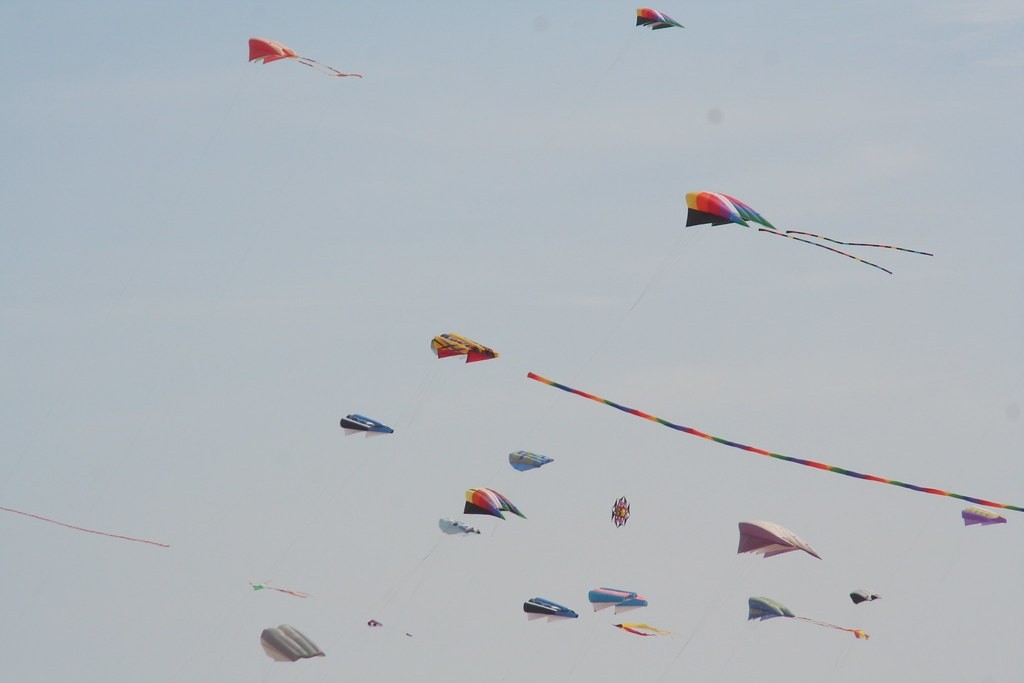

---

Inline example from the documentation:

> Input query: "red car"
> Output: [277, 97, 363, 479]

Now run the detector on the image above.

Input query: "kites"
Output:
[368, 618, 413, 638]
[250, 580, 307, 598]
[463, 488, 526, 520]
[961, 505, 1007, 527]
[249, 37, 362, 78]
[429, 331, 1024, 514]
[509, 451, 553, 472]
[748, 595, 869, 639]
[738, 520, 822, 561]
[588, 585, 647, 615]
[636, 8, 685, 30]
[611, 497, 630, 527]
[851, 589, 881, 605]
[613, 622, 671, 637]
[523, 597, 578, 623]
[686, 191, 934, 274]
[340, 413, 393, 438]
[260, 624, 326, 662]
[439, 518, 481, 537]
